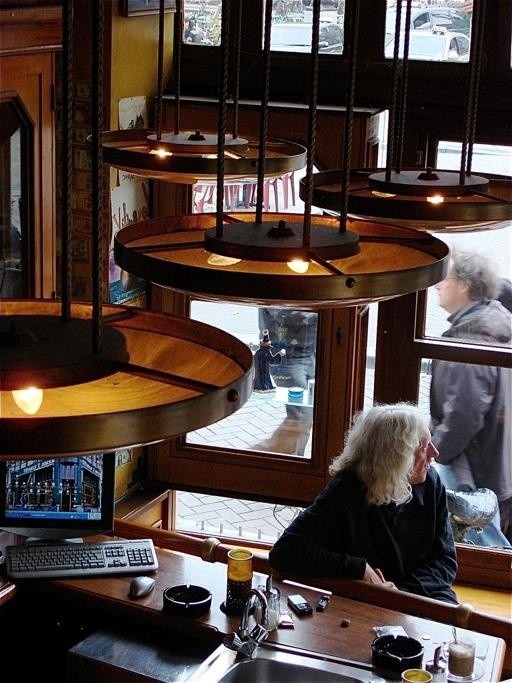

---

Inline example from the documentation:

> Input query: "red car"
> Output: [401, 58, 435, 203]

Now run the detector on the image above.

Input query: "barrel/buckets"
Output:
[288, 386, 303, 404]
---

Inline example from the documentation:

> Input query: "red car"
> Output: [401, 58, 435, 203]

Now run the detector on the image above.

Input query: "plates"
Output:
[444, 661, 485, 683]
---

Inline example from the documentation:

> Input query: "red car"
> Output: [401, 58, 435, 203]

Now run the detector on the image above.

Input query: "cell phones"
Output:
[287, 594, 313, 616]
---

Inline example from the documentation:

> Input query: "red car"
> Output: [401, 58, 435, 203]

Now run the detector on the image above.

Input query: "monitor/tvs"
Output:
[0, 451, 115, 545]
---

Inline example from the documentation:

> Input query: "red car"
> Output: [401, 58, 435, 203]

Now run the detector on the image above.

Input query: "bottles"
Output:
[253, 582, 281, 632]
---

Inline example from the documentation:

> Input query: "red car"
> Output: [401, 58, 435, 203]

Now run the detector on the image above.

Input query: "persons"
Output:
[425, 239, 510, 544]
[259, 305, 318, 388]
[493, 274, 511, 313]
[184, 19, 196, 37]
[265, 400, 459, 606]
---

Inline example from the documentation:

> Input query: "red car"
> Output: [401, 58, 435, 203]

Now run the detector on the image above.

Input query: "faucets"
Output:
[240, 589, 270, 630]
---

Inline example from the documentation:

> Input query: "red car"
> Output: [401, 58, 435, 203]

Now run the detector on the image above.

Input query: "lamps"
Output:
[294, 0, 511, 232]
[114, 0, 449, 312]
[0, 0, 253, 449]
[83, 1, 309, 187]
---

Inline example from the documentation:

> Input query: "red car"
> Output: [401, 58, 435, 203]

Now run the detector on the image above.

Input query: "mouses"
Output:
[130, 576, 156, 599]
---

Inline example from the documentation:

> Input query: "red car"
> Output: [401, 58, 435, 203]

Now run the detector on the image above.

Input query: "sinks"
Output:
[196, 643, 403, 683]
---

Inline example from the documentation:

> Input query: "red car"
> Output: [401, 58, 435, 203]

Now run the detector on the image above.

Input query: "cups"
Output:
[224, 547, 254, 614]
[447, 638, 477, 677]
[399, 668, 434, 683]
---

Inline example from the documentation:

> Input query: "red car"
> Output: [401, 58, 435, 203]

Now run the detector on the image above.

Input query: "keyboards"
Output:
[5, 539, 159, 579]
